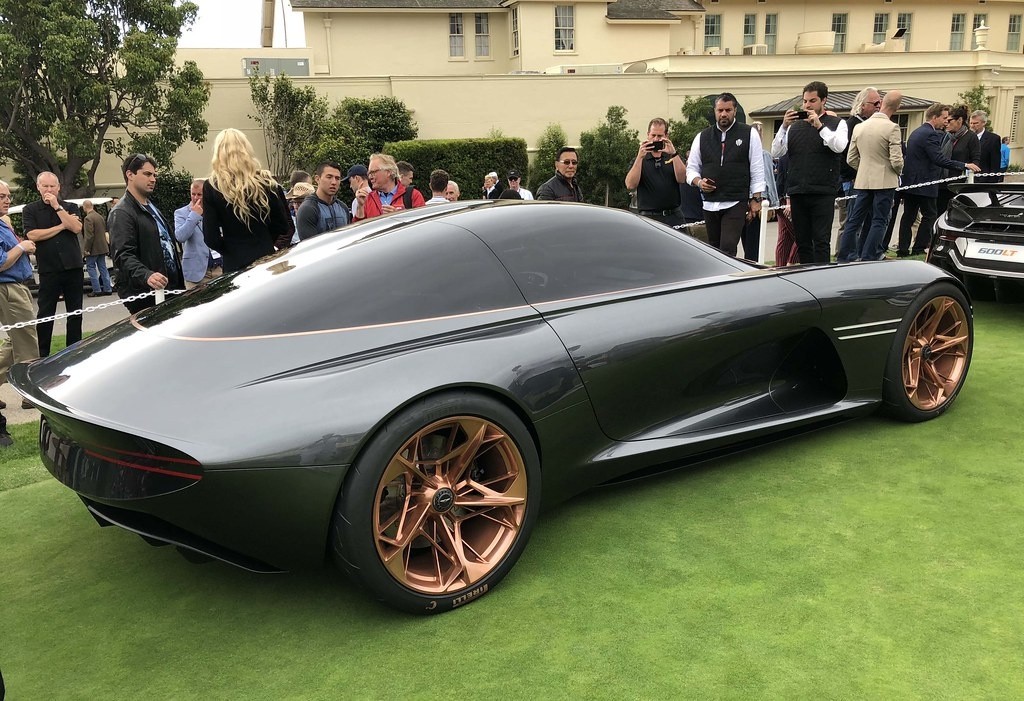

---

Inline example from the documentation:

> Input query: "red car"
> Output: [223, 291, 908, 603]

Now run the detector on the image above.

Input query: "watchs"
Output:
[752, 197, 762, 202]
[55, 205, 63, 212]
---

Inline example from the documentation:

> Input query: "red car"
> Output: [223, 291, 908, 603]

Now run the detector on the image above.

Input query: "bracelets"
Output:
[818, 123, 826, 132]
[17, 244, 25, 251]
[671, 151, 679, 158]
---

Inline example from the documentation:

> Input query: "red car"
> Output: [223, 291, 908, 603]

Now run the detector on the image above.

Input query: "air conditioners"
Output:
[743, 43, 768, 55]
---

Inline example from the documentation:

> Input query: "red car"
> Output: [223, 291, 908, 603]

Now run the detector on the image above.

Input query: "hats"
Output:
[285, 182, 315, 199]
[507, 170, 521, 178]
[341, 165, 367, 183]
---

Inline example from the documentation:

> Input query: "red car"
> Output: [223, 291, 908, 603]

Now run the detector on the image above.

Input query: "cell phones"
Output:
[793, 111, 809, 118]
[645, 141, 663, 150]
[705, 179, 715, 186]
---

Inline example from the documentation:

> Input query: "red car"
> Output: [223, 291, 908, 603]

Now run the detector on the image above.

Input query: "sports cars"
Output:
[8, 199, 975, 615]
[927, 179, 1023, 303]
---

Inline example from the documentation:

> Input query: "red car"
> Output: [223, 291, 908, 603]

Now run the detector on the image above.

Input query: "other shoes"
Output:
[103, 292, 112, 295]
[0, 433, 13, 446]
[87, 292, 102, 297]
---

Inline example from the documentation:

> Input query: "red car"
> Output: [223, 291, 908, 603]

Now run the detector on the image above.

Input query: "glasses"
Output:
[948, 118, 959, 123]
[368, 169, 391, 176]
[290, 201, 303, 206]
[509, 177, 518, 181]
[864, 101, 882, 106]
[128, 154, 150, 170]
[558, 161, 578, 165]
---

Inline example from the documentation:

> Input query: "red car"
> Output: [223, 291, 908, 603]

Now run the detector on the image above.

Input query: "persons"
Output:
[22, 172, 84, 357]
[482, 170, 534, 200]
[771, 81, 906, 267]
[1001, 137, 1010, 181]
[426, 170, 460, 204]
[0, 181, 40, 409]
[536, 147, 584, 202]
[342, 154, 426, 222]
[83, 197, 119, 297]
[625, 92, 779, 261]
[896, 103, 1001, 257]
[107, 155, 187, 315]
[174, 129, 296, 291]
[286, 162, 350, 241]
